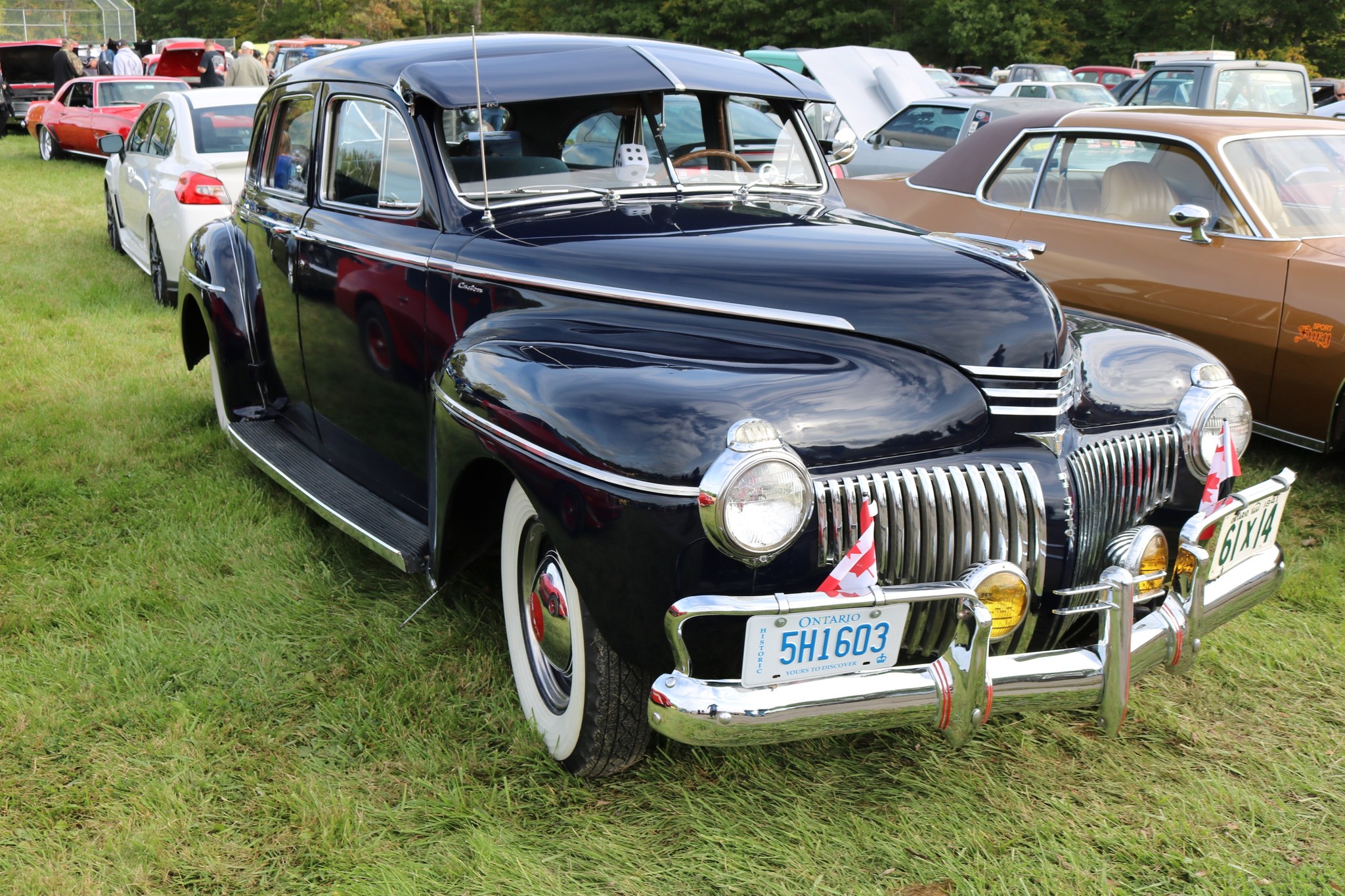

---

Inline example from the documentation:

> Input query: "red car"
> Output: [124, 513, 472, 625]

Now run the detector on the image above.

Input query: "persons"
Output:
[113, 39, 144, 98]
[229, 46, 274, 73]
[0, 76, 9, 139]
[225, 41, 269, 87]
[83, 44, 114, 75]
[197, 39, 226, 88]
[274, 130, 292, 190]
[947, 67, 962, 72]
[53, 37, 85, 106]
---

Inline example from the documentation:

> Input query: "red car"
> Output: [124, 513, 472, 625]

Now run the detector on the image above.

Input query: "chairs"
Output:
[1100, 161, 1180, 228]
[1214, 168, 1306, 240]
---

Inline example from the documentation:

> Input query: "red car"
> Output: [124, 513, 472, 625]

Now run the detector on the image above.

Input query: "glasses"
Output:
[1336, 92, 1345, 97]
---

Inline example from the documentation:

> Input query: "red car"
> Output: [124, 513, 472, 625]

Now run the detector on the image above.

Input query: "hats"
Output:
[61, 36, 77, 46]
[88, 44, 93, 48]
[302, 53, 309, 60]
[86, 56, 95, 67]
[100, 43, 106, 47]
[241, 41, 255, 50]
[253, 49, 263, 57]
[116, 39, 129, 46]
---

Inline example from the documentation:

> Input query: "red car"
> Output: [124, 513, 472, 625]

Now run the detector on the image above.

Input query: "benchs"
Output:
[450, 155, 569, 183]
[986, 169, 1105, 218]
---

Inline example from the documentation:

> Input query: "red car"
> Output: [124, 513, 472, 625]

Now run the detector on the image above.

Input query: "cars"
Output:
[178, 32, 1297, 781]
[0, 37, 234, 138]
[257, 38, 1345, 468]
[21, 76, 253, 162]
[97, 89, 383, 310]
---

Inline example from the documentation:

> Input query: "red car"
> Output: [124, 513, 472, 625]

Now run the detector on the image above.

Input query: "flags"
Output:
[814, 497, 878, 592]
[1198, 424, 1242, 540]
